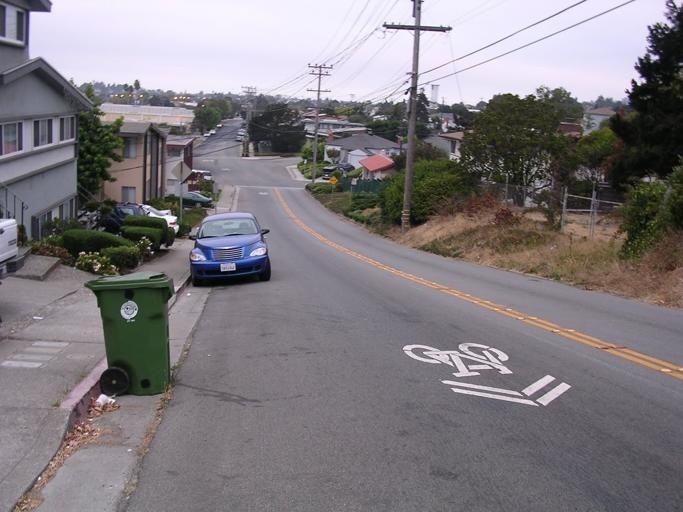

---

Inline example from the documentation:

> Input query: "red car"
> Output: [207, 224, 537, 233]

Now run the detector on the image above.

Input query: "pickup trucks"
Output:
[0, 216, 25, 285]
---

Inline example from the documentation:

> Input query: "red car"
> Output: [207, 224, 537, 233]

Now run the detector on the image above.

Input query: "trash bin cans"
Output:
[85, 272, 176, 396]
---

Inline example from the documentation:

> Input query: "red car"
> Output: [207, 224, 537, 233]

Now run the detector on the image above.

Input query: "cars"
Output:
[202, 123, 224, 138]
[92, 189, 213, 241]
[337, 162, 355, 171]
[186, 210, 272, 286]
[235, 127, 245, 142]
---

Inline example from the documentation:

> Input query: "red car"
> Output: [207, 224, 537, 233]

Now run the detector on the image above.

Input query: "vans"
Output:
[320, 166, 343, 181]
